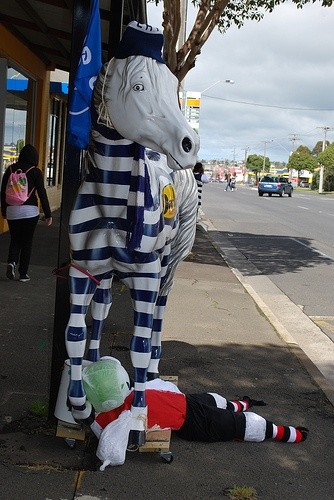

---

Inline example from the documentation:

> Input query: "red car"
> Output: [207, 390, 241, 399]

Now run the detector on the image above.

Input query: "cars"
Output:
[3, 144, 19, 160]
[47, 158, 53, 180]
[258, 174, 294, 198]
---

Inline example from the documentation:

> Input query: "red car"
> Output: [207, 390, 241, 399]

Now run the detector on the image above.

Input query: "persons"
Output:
[0, 145, 52, 281]
[224, 172, 238, 192]
[192, 162, 209, 223]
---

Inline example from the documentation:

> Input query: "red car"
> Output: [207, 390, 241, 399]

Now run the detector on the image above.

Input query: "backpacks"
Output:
[5, 165, 36, 206]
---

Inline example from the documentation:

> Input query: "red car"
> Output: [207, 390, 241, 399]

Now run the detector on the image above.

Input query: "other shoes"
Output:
[6, 261, 16, 280]
[19, 273, 30, 281]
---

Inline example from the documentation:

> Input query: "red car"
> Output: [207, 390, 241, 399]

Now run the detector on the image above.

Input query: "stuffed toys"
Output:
[81, 356, 309, 444]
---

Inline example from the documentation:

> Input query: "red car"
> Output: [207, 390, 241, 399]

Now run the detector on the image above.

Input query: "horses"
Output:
[64, 20, 199, 450]
